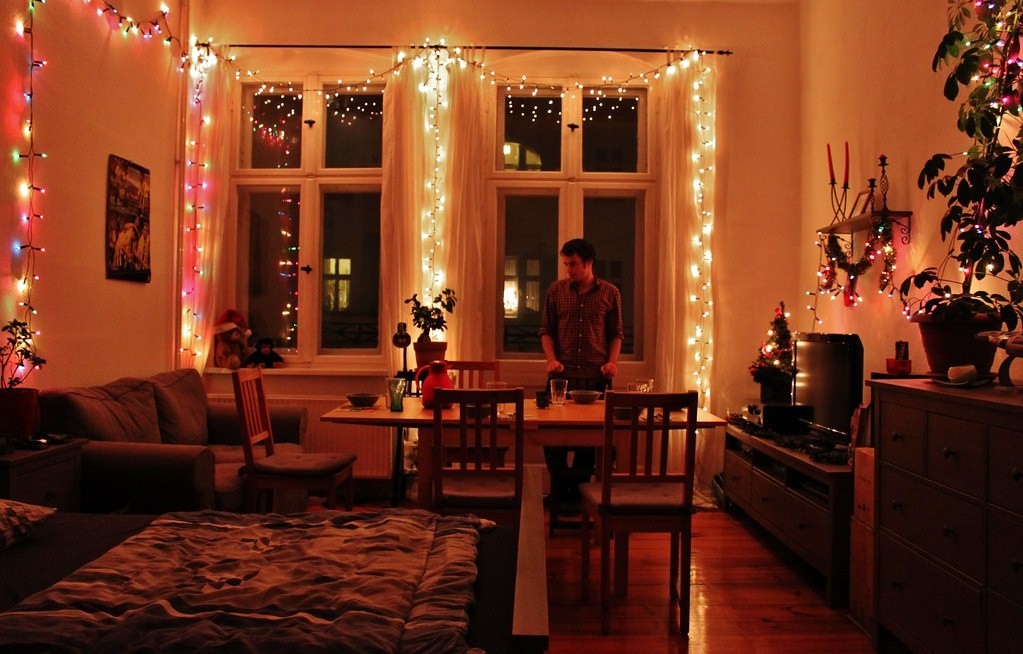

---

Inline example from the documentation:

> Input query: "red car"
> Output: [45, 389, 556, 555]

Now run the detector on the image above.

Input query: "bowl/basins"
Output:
[345, 393, 380, 407]
[568, 390, 603, 404]
[614, 407, 644, 420]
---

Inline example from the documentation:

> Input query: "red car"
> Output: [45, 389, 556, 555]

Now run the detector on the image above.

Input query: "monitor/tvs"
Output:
[793, 332, 864, 451]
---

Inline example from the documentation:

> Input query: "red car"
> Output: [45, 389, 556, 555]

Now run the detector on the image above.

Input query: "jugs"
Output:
[415, 361, 456, 409]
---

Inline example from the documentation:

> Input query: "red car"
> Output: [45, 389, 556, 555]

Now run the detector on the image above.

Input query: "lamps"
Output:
[392, 322, 414, 388]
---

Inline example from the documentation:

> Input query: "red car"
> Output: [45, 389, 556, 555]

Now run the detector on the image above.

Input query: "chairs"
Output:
[232, 367, 359, 510]
[431, 387, 524, 521]
[582, 390, 697, 636]
[418, 361, 510, 467]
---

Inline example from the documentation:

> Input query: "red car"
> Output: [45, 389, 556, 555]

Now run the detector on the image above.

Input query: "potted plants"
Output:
[409, 289, 460, 389]
[900, 0, 1023, 383]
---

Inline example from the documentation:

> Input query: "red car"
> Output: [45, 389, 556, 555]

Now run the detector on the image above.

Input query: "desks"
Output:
[324, 394, 732, 537]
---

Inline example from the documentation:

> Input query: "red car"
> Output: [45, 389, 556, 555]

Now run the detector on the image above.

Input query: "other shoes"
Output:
[542, 496, 576, 517]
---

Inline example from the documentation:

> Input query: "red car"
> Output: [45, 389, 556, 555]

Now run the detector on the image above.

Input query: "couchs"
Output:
[0, 365, 307, 611]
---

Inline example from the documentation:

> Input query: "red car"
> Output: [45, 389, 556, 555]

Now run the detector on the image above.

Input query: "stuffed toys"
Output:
[213, 310, 287, 369]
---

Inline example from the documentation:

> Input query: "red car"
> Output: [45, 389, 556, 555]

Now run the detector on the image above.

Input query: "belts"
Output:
[566, 369, 595, 378]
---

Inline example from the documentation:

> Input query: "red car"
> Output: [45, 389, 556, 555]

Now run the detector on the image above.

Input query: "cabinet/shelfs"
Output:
[718, 424, 855, 574]
[866, 377, 1023, 654]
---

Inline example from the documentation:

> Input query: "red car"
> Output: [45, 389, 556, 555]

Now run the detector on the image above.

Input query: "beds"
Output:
[0, 510, 500, 654]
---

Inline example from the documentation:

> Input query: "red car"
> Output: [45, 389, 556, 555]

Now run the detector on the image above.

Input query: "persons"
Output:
[540, 238, 625, 517]
[110, 167, 151, 269]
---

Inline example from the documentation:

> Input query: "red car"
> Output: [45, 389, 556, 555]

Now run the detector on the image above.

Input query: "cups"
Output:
[628, 378, 654, 414]
[384, 378, 406, 412]
[551, 379, 568, 405]
[487, 382, 506, 413]
[536, 391, 550, 408]
